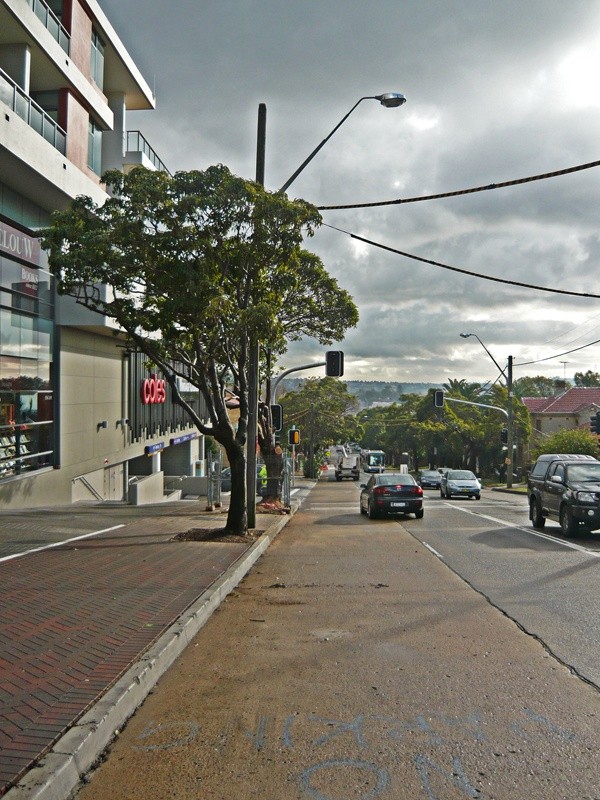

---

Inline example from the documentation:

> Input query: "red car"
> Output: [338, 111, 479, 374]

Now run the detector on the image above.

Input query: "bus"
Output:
[334, 450, 361, 481]
[362, 449, 386, 474]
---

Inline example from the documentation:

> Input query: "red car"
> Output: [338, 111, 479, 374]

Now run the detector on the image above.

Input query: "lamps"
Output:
[98, 421, 108, 428]
[117, 419, 127, 428]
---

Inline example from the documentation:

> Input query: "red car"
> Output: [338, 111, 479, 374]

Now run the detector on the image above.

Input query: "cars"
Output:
[437, 466, 452, 477]
[439, 469, 482, 500]
[335, 440, 363, 455]
[414, 469, 442, 490]
[358, 472, 425, 518]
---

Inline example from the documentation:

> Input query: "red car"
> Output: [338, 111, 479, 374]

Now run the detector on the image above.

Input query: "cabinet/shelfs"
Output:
[0, 390, 35, 478]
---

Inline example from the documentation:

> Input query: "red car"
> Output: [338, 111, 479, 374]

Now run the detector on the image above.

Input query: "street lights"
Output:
[245, 91, 409, 525]
[459, 332, 514, 491]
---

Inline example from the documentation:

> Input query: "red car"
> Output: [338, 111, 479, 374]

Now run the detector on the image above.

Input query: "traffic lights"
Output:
[597, 438, 600, 447]
[435, 390, 444, 407]
[325, 351, 340, 377]
[500, 428, 507, 445]
[590, 411, 600, 435]
[269, 403, 281, 433]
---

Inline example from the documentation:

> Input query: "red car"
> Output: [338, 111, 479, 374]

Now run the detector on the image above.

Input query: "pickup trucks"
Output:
[526, 454, 599, 537]
